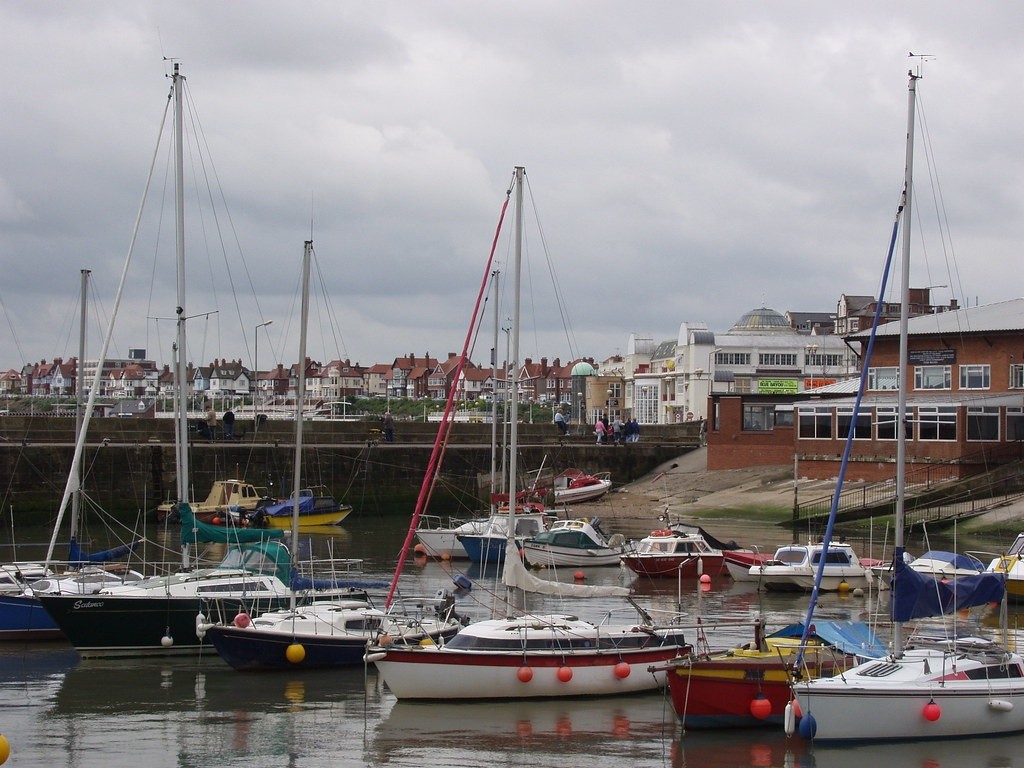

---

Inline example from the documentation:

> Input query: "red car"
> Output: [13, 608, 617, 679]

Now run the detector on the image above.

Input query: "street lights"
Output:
[529, 397, 534, 424]
[475, 402, 478, 423]
[423, 395, 428, 423]
[252, 321, 273, 436]
[550, 394, 556, 424]
[606, 390, 613, 425]
[577, 393, 583, 425]
[707, 348, 722, 398]
[805, 344, 819, 392]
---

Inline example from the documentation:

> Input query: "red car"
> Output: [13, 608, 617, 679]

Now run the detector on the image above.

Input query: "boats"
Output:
[412, 455, 1024, 604]
[627, 614, 889, 733]
[154, 460, 358, 532]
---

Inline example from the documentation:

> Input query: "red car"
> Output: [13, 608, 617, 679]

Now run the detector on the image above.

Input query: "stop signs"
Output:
[687, 411, 694, 419]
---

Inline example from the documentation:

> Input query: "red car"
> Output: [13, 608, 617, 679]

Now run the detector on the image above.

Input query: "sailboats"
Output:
[189, 219, 479, 677]
[1, 55, 398, 660]
[774, 50, 1024, 742]
[352, 164, 702, 703]
[0, 267, 163, 644]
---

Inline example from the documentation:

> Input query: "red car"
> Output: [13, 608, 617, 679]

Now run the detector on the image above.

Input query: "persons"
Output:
[384, 412, 393, 441]
[625, 418, 632, 442]
[555, 409, 570, 436]
[595, 419, 607, 444]
[632, 418, 640, 442]
[204, 406, 217, 444]
[700, 418, 706, 446]
[223, 408, 234, 440]
[602, 414, 608, 444]
[609, 424, 613, 442]
[613, 416, 625, 442]
